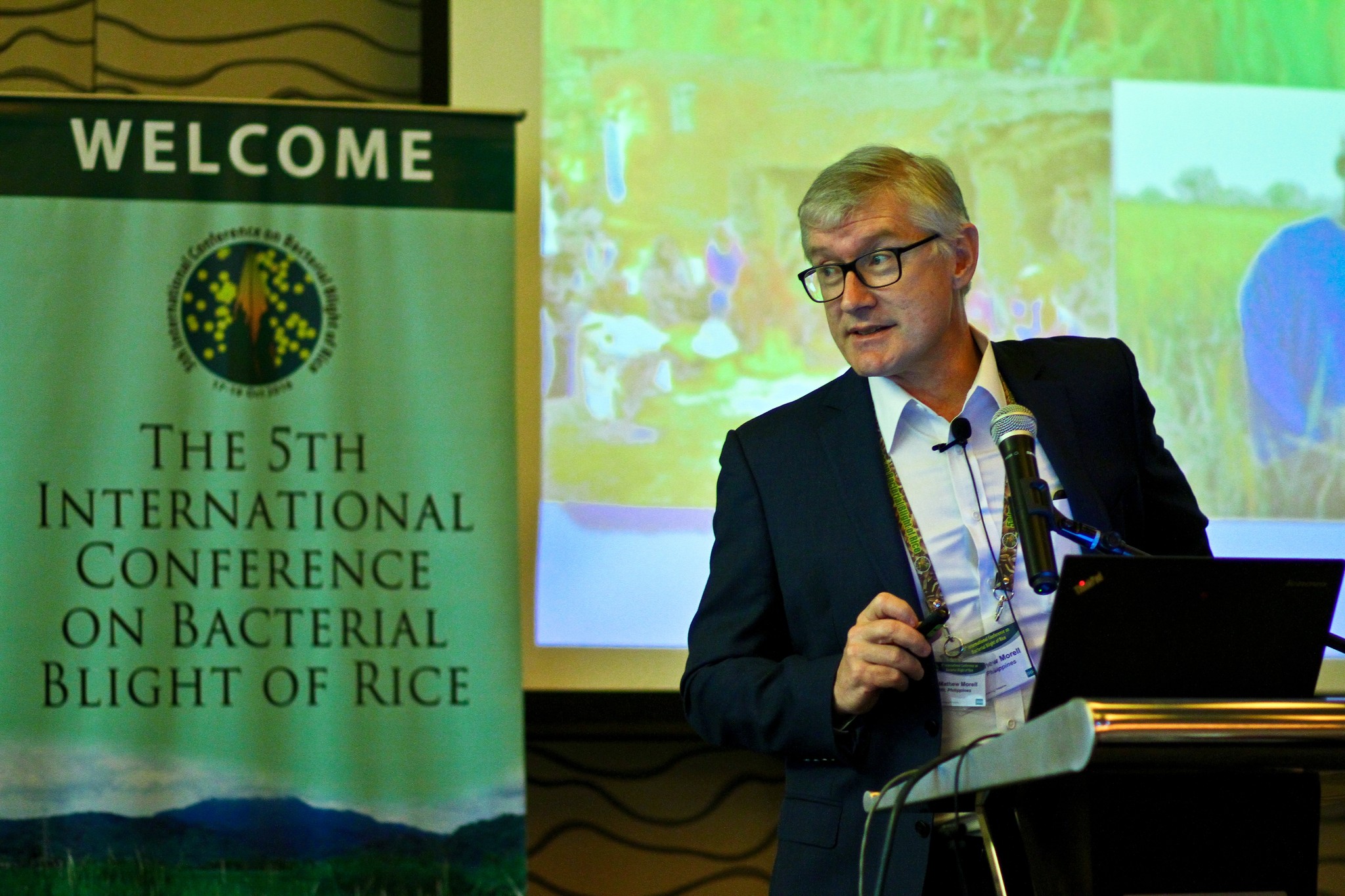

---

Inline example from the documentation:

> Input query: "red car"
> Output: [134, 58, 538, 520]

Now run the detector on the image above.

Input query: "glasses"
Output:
[797, 232, 942, 303]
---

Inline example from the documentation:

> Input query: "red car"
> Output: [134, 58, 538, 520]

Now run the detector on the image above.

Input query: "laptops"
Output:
[1025, 555, 1345, 723]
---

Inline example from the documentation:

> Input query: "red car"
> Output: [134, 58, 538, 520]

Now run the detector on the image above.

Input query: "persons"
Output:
[679, 147, 1214, 896]
[1240, 138, 1345, 472]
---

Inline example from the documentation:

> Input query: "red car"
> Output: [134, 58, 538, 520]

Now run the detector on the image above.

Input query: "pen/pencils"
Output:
[916, 606, 949, 639]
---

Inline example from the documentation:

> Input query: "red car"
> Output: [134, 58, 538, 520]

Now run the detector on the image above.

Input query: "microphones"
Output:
[951, 417, 972, 446]
[990, 403, 1059, 595]
